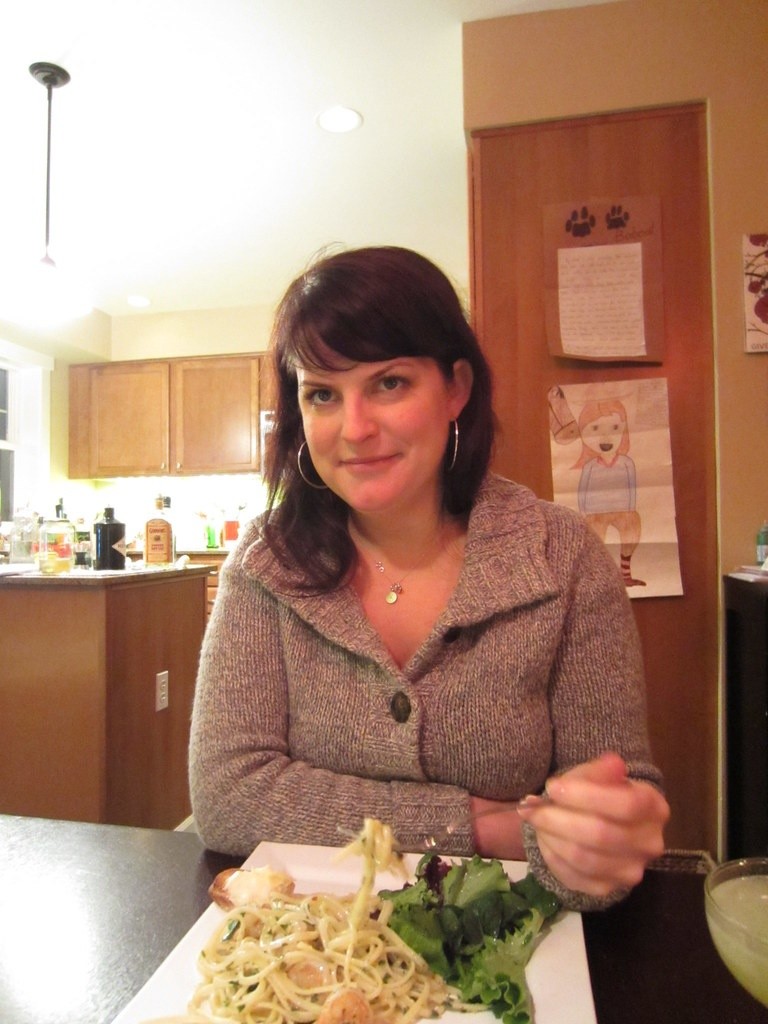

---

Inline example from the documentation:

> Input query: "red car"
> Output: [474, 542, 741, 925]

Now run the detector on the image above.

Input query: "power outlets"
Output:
[155, 670, 169, 711]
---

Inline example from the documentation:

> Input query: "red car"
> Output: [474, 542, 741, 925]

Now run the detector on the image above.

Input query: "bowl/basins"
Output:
[703, 857, 768, 1007]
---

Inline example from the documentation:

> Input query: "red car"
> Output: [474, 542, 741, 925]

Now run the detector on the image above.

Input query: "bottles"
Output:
[40, 520, 75, 575]
[755, 519, 768, 565]
[76, 517, 92, 568]
[143, 495, 175, 567]
[93, 507, 125, 570]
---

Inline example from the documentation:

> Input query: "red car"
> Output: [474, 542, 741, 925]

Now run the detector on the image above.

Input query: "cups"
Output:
[74, 541, 89, 568]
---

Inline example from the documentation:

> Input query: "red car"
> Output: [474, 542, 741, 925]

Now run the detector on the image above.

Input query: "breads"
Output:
[208, 868, 295, 910]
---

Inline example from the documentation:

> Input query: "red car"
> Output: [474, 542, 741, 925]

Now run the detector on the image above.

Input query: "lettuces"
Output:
[370, 854, 563, 1024]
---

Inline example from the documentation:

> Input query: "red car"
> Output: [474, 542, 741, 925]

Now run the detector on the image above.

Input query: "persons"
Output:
[188, 246, 670, 913]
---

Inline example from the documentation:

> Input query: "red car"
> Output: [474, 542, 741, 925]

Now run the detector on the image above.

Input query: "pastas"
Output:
[133, 818, 489, 1024]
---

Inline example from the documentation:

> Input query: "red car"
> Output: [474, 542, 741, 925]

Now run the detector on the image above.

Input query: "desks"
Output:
[0, 812, 768, 1024]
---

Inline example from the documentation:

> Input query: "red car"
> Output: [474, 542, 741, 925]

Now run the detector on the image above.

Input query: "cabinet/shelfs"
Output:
[0, 551, 234, 831]
[67, 351, 278, 480]
[724, 574, 768, 860]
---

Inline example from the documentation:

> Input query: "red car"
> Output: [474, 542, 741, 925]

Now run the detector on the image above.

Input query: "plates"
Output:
[109, 841, 597, 1024]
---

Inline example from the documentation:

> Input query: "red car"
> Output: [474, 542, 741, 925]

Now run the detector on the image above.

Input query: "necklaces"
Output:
[356, 538, 443, 604]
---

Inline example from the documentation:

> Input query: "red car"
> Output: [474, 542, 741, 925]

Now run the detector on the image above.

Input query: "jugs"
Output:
[10, 514, 34, 563]
